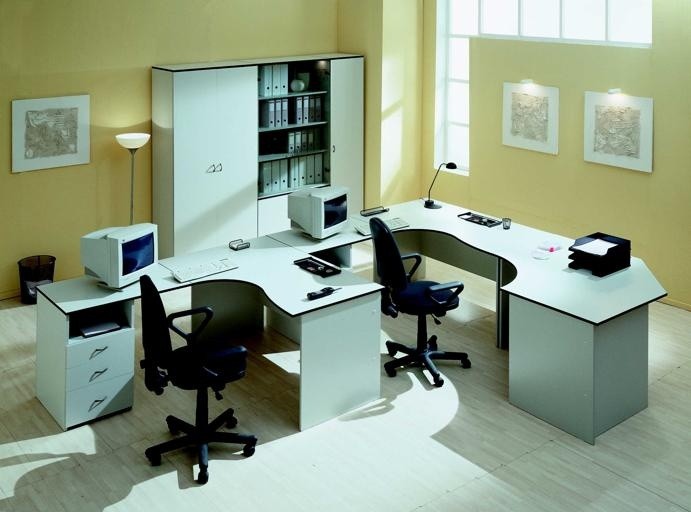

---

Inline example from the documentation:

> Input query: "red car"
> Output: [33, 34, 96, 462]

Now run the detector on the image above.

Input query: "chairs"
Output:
[367, 216, 472, 389]
[138, 273, 257, 486]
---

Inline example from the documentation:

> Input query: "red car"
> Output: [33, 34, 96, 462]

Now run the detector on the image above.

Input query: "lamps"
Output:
[115, 131, 152, 228]
[422, 162, 456, 209]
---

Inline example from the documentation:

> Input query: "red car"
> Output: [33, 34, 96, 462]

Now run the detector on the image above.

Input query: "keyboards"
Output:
[172, 257, 240, 283]
[354, 217, 409, 236]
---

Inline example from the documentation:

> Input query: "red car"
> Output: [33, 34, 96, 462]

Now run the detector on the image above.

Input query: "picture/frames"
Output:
[500, 80, 559, 157]
[9, 94, 93, 174]
[583, 90, 654, 175]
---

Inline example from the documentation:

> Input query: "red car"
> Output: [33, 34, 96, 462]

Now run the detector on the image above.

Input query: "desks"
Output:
[266, 196, 668, 449]
[33, 237, 383, 432]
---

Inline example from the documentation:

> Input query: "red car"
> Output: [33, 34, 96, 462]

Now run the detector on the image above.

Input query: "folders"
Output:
[281, 127, 325, 153]
[263, 95, 328, 128]
[259, 153, 326, 194]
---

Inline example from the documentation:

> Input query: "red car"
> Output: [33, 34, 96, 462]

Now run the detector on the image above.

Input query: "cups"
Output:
[502, 217, 511, 230]
[297, 71, 310, 89]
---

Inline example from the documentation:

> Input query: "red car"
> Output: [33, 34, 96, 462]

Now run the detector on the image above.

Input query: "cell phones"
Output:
[307, 286, 342, 300]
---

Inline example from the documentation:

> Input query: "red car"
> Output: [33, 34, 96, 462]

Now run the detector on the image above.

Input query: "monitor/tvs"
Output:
[80, 223, 158, 292]
[288, 185, 350, 242]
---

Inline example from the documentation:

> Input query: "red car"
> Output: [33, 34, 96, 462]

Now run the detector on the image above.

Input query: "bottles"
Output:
[290, 79, 306, 92]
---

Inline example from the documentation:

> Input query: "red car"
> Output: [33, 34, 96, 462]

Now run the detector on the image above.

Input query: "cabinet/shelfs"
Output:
[258, 182, 328, 236]
[253, 57, 332, 201]
[331, 52, 366, 224]
[151, 57, 258, 257]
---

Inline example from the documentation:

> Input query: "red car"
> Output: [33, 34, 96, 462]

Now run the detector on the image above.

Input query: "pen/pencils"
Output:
[259, 63, 291, 97]
